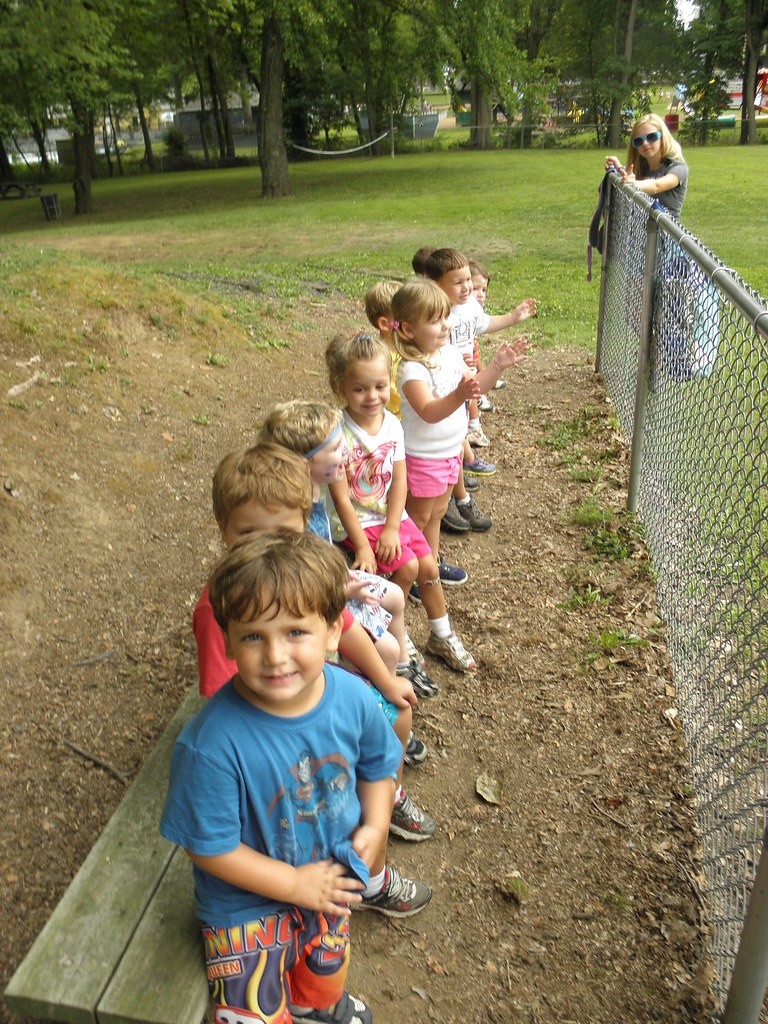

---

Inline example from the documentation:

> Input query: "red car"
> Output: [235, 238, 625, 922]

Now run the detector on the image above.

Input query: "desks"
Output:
[0, 181, 38, 200]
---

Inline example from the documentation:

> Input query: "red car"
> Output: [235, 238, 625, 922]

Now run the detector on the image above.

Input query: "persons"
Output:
[659, 89, 664, 101]
[192, 441, 437, 919]
[666, 89, 670, 102]
[364, 279, 492, 532]
[260, 397, 439, 767]
[392, 274, 529, 603]
[412, 243, 493, 416]
[320, 330, 476, 673]
[426, 252, 537, 492]
[157, 526, 406, 1024]
[467, 257, 505, 410]
[605, 113, 689, 383]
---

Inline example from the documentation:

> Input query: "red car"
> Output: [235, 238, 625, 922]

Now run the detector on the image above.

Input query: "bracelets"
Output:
[494, 360, 502, 371]
[618, 167, 625, 170]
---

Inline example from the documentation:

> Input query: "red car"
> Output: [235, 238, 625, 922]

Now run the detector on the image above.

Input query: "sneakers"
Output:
[405, 632, 425, 669]
[396, 660, 440, 697]
[402, 733, 427, 765]
[466, 426, 490, 447]
[289, 991, 372, 1024]
[437, 554, 468, 586]
[464, 475, 480, 491]
[442, 500, 472, 531]
[455, 499, 493, 532]
[462, 453, 497, 477]
[389, 790, 437, 843]
[407, 583, 423, 603]
[348, 863, 433, 918]
[426, 631, 477, 671]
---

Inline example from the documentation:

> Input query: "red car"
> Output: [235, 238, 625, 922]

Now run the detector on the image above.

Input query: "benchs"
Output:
[0, 477, 388, 1024]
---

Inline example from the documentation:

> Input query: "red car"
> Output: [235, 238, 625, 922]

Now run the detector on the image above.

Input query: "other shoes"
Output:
[477, 395, 493, 412]
[493, 381, 507, 390]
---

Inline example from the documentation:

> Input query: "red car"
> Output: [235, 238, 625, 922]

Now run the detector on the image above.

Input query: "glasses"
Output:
[632, 132, 662, 149]
